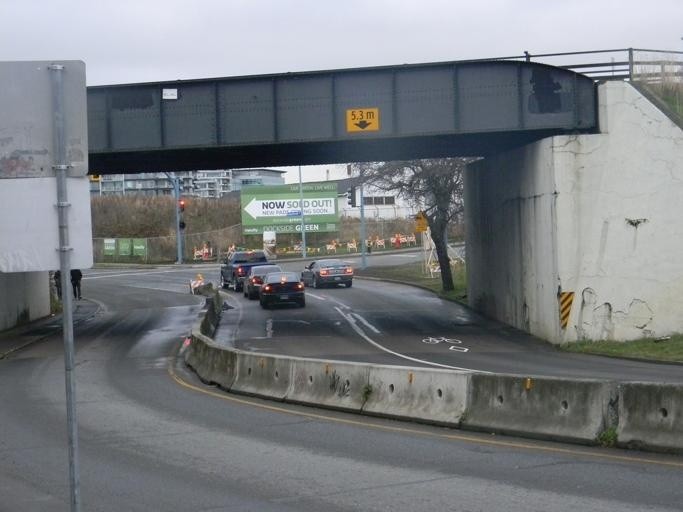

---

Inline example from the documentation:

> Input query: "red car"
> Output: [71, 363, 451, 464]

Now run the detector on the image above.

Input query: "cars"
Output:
[219, 251, 353, 309]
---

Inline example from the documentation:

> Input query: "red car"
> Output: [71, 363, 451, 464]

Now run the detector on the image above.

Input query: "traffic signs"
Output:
[239, 182, 337, 236]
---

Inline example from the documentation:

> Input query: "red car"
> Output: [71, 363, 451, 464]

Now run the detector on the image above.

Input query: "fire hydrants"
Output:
[395, 235, 401, 249]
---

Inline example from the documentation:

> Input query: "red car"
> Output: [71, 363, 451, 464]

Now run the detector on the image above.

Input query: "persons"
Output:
[53, 269, 61, 297]
[68, 269, 82, 300]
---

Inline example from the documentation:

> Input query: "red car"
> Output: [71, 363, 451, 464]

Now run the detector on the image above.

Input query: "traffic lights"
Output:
[179, 221, 184, 229]
[347, 185, 355, 207]
[178, 200, 185, 213]
